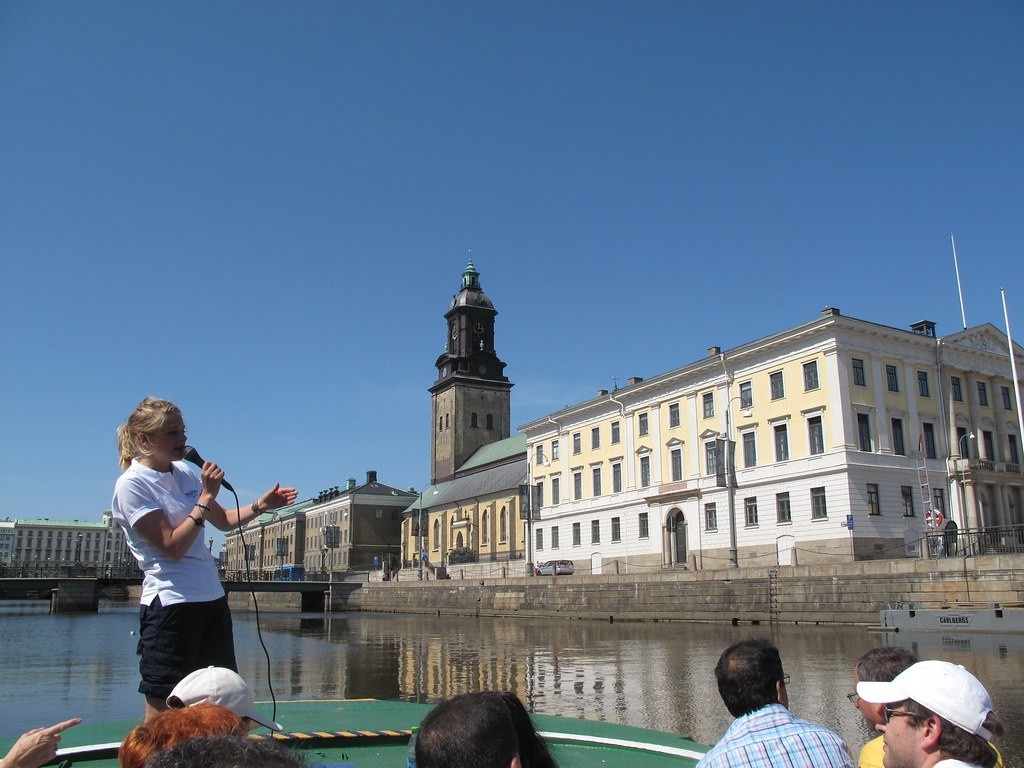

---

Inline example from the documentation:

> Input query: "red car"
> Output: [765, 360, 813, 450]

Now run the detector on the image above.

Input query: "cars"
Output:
[537, 560, 574, 575]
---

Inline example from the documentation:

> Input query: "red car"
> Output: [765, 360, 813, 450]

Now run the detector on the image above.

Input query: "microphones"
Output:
[182, 446, 234, 492]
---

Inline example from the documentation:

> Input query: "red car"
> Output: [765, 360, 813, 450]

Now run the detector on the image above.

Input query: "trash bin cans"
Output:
[435, 567, 446, 579]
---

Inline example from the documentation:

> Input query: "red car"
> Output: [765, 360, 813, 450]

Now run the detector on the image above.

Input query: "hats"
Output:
[857, 660, 996, 741]
[167, 665, 284, 731]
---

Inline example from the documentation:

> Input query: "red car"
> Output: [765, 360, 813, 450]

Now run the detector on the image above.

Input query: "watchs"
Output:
[187, 514, 205, 528]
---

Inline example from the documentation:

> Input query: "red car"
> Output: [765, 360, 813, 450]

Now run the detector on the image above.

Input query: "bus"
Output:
[274, 565, 305, 581]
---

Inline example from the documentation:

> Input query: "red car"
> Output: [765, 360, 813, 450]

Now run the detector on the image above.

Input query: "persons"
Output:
[846, 647, 1006, 768]
[857, 660, 1004, 768]
[111, 395, 298, 722]
[696, 640, 856, 768]
[118, 666, 560, 768]
[0, 718, 83, 768]
[534, 561, 540, 575]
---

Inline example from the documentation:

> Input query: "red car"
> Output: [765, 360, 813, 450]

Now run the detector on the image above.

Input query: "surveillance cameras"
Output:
[970, 435, 975, 440]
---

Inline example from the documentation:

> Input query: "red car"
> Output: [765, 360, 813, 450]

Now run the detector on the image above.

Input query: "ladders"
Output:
[769, 567, 778, 624]
[915, 457, 939, 559]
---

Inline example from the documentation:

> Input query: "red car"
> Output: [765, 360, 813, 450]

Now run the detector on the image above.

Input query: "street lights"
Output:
[21, 533, 131, 579]
[209, 537, 214, 552]
[418, 483, 439, 580]
[725, 397, 752, 568]
[959, 431, 975, 557]
[280, 522, 296, 580]
[248, 530, 261, 571]
[525, 453, 550, 575]
[329, 506, 347, 583]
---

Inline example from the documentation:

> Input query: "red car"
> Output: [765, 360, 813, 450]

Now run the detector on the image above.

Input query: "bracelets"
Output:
[195, 503, 212, 512]
[251, 500, 262, 516]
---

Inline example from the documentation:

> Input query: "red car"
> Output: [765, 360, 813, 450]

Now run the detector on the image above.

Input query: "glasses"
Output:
[847, 692, 861, 703]
[884, 706, 924, 723]
[780, 673, 790, 684]
